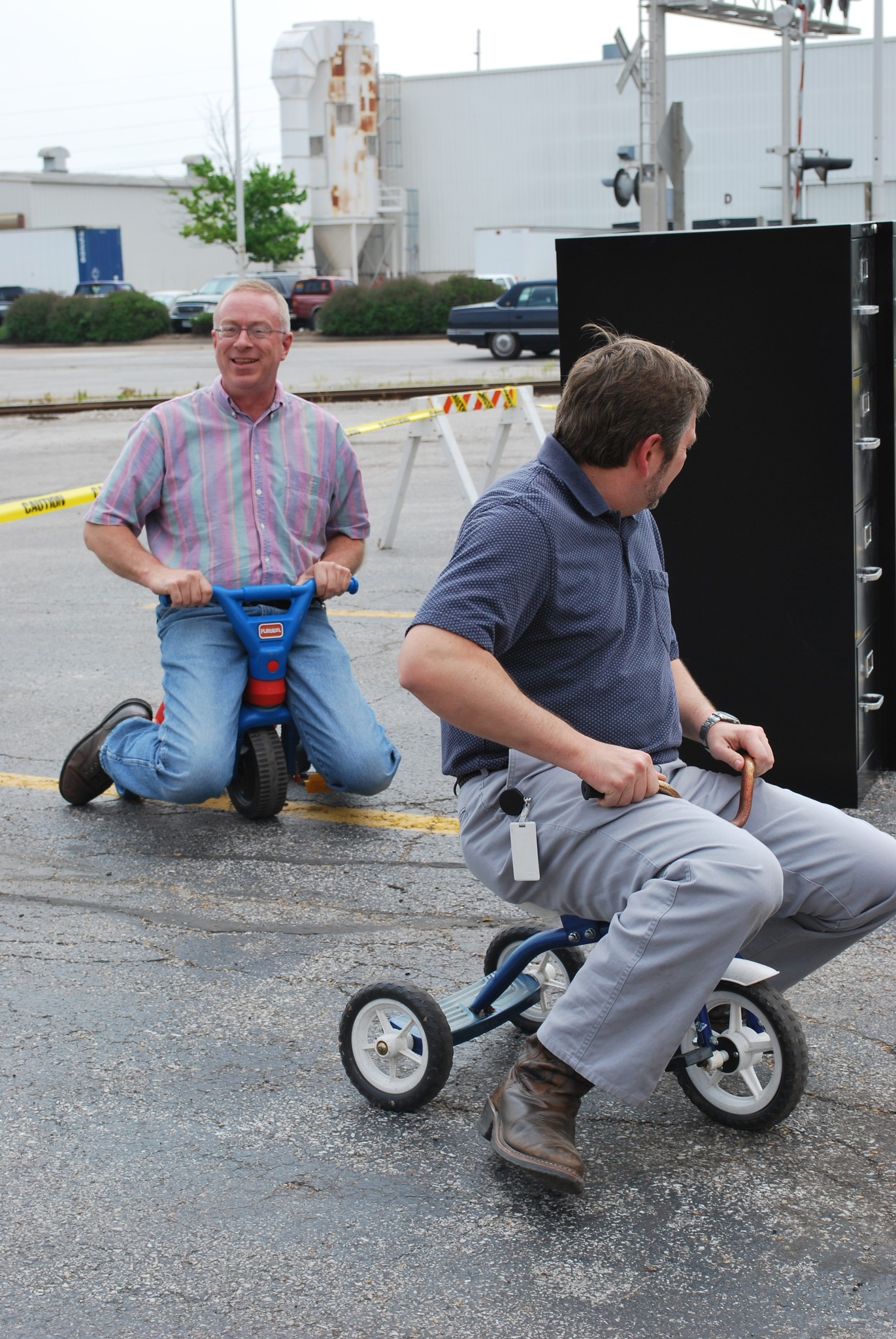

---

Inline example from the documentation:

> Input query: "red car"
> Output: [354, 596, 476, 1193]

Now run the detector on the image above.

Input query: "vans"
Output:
[289, 275, 361, 331]
[0, 286, 48, 322]
[169, 270, 299, 333]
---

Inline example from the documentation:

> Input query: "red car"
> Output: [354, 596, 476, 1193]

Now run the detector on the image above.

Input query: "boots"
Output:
[475, 1031, 596, 1195]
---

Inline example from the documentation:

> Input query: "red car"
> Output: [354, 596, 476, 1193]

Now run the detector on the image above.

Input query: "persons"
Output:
[397, 319, 896, 1196]
[56, 276, 402, 806]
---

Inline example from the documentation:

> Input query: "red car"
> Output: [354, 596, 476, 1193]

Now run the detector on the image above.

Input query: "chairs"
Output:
[523, 289, 549, 306]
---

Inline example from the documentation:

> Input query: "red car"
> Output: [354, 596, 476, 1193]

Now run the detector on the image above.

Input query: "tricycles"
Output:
[154, 582, 359, 821]
[340, 750, 809, 1135]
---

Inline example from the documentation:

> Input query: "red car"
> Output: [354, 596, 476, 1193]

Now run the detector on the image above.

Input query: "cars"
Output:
[445, 282, 564, 362]
[71, 280, 136, 301]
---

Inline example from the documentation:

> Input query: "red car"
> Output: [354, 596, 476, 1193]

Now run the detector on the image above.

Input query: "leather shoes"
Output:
[58, 697, 153, 805]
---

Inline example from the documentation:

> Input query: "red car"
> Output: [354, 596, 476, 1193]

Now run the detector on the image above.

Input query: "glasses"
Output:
[214, 324, 287, 342]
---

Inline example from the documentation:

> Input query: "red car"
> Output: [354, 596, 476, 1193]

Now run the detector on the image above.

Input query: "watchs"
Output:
[699, 711, 741, 753]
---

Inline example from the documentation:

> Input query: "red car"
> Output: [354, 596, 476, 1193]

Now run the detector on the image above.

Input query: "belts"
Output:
[457, 761, 507, 789]
